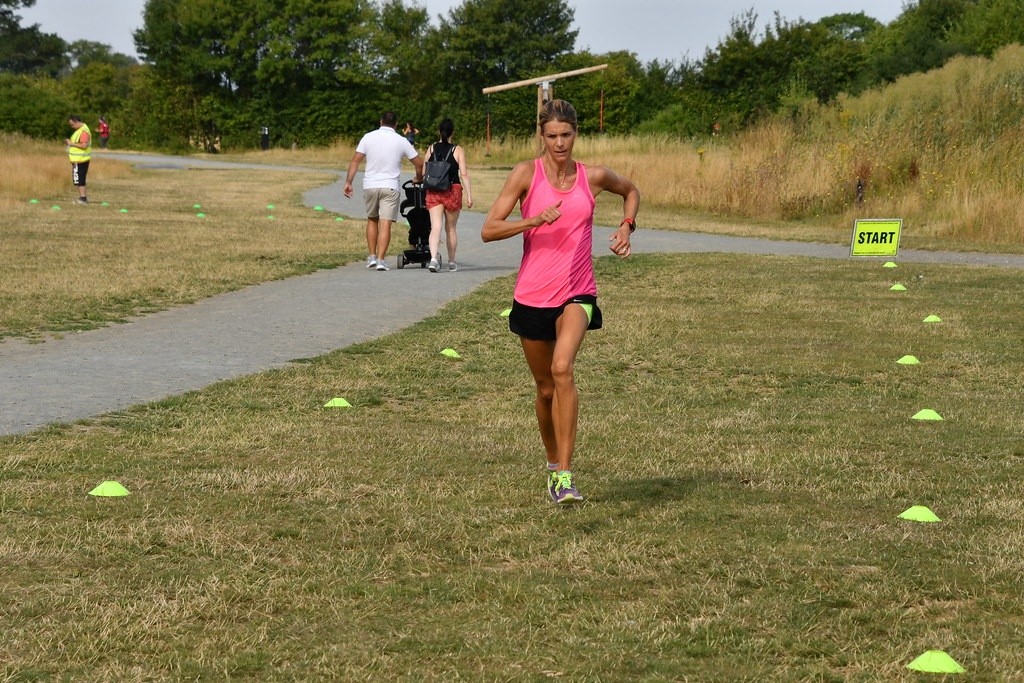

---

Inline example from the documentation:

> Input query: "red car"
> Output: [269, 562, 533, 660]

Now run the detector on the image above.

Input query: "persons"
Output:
[95, 117, 111, 150]
[65, 115, 91, 206]
[480, 99, 641, 506]
[402, 120, 420, 163]
[342, 111, 424, 271]
[422, 118, 473, 272]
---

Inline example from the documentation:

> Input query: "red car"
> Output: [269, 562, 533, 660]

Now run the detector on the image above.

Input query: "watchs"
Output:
[620, 218, 637, 234]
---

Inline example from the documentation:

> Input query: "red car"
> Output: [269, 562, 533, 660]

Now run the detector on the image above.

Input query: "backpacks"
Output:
[423, 141, 457, 192]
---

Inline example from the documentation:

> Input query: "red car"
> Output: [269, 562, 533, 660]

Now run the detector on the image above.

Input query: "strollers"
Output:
[396, 178, 443, 269]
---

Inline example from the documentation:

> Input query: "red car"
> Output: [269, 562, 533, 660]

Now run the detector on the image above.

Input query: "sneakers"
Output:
[545, 460, 562, 504]
[428, 260, 442, 272]
[376, 259, 392, 271]
[366, 255, 378, 268]
[448, 259, 458, 272]
[550, 471, 583, 503]
[72, 198, 90, 205]
